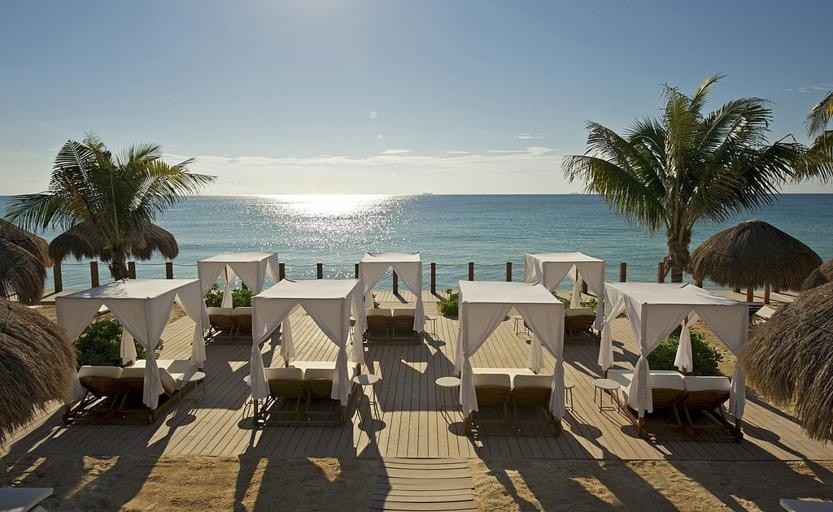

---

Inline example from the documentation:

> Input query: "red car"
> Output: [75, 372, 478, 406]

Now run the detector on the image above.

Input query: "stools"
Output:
[512, 315, 531, 338]
[242, 374, 255, 419]
[592, 377, 623, 414]
[353, 371, 378, 407]
[175, 371, 207, 409]
[562, 381, 578, 412]
[436, 375, 463, 412]
[423, 315, 439, 337]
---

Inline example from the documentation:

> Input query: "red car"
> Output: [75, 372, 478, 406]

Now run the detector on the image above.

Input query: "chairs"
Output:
[260, 357, 361, 430]
[363, 308, 423, 350]
[608, 363, 739, 444]
[467, 363, 561, 440]
[205, 306, 258, 344]
[64, 355, 202, 428]
[564, 308, 603, 342]
